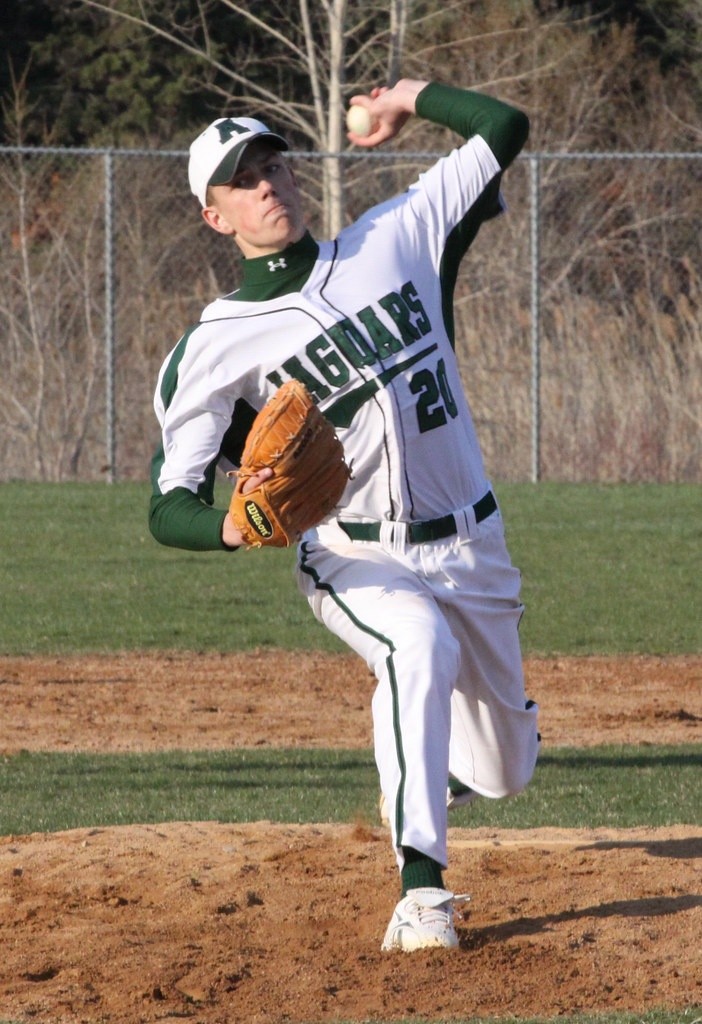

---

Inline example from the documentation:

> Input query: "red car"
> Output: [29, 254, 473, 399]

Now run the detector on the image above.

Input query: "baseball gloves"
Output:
[224, 375, 359, 555]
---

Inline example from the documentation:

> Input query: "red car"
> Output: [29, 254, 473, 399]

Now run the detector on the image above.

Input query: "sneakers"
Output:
[380, 886, 472, 951]
[379, 786, 478, 827]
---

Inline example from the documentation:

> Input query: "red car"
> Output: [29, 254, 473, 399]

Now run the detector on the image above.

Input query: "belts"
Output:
[338, 492, 498, 545]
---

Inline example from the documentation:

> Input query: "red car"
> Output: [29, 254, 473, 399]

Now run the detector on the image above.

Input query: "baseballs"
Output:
[345, 104, 381, 137]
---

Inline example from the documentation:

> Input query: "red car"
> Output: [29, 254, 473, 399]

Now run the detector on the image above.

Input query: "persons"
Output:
[147, 78, 541, 955]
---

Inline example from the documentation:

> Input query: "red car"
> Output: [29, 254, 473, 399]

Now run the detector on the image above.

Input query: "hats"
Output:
[188, 116, 289, 209]
